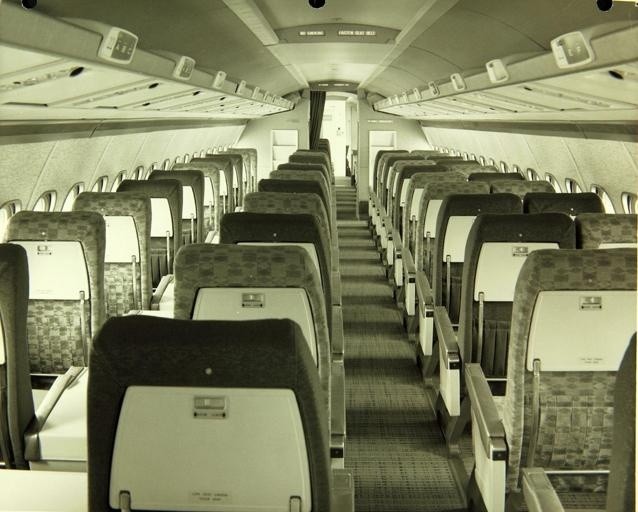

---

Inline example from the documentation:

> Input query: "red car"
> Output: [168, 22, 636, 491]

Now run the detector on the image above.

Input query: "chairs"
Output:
[0, 149, 353, 512]
[368, 150, 638, 512]
